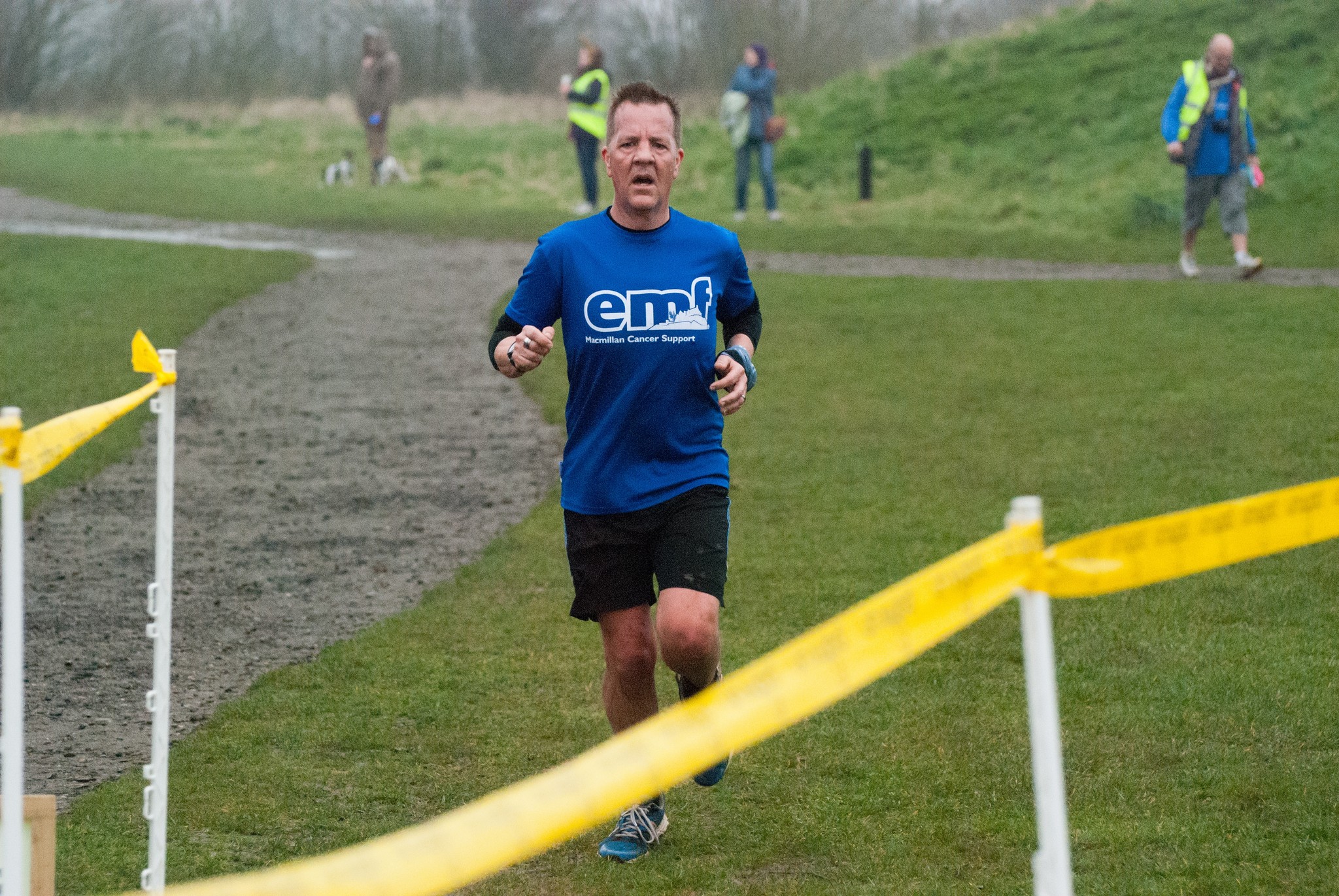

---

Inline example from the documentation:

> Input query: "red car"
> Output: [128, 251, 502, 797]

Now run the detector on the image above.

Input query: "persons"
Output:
[354, 26, 402, 185]
[489, 82, 763, 863]
[1162, 32, 1263, 278]
[720, 42, 783, 222]
[560, 42, 613, 216]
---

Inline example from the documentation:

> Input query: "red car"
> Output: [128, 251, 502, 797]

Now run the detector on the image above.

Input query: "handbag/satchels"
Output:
[765, 116, 790, 139]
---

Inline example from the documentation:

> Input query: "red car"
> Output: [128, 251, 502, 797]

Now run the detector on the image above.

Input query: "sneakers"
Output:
[598, 789, 669, 865]
[675, 665, 733, 786]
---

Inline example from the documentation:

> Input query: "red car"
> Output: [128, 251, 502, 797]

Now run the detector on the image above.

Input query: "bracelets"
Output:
[715, 346, 757, 393]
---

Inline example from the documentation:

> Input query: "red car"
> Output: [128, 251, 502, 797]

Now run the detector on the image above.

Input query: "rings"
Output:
[523, 337, 531, 350]
[742, 394, 746, 403]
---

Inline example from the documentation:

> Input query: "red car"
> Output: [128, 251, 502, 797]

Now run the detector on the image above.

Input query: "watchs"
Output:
[507, 341, 516, 367]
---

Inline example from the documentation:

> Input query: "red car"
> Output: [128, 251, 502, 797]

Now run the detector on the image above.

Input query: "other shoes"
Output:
[1182, 253, 1201, 277]
[767, 210, 781, 222]
[1236, 255, 1262, 279]
[734, 212, 747, 223]
